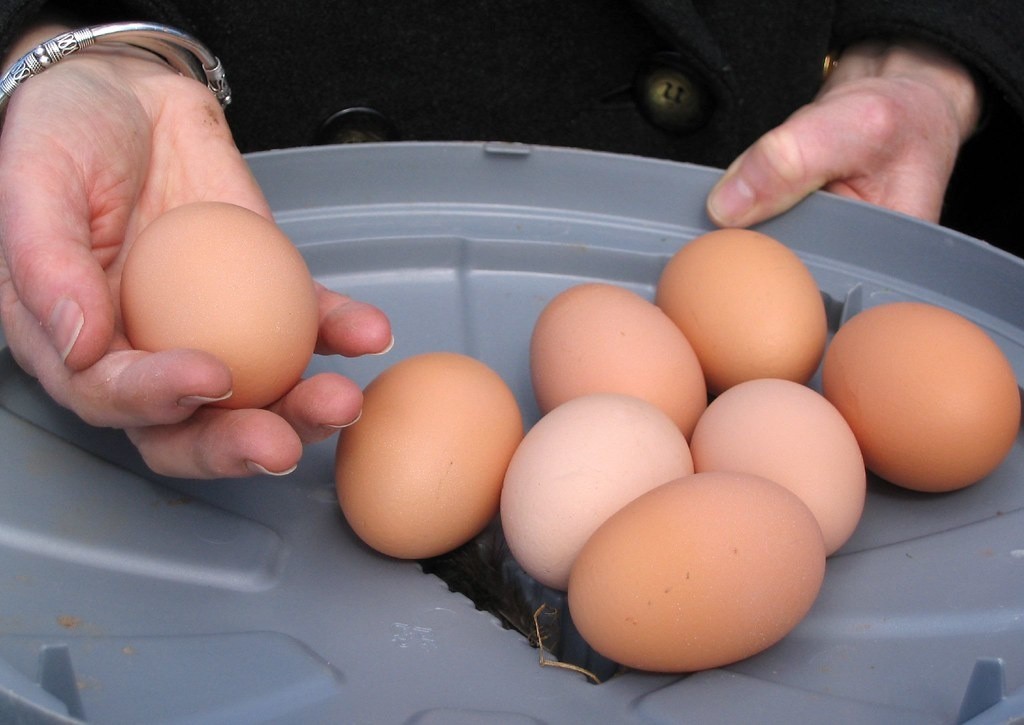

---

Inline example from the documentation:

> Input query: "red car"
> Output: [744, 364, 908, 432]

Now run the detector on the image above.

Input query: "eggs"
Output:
[336, 226, 1021, 673]
[122, 203, 318, 408]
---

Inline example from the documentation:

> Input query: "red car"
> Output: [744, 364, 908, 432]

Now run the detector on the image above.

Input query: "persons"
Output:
[0, 0, 1024, 481]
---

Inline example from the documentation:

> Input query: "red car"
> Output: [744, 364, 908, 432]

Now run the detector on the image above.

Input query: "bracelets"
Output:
[0, 21, 231, 117]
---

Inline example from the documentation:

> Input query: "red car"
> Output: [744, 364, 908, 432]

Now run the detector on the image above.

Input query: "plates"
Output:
[0, 137, 1024, 725]
